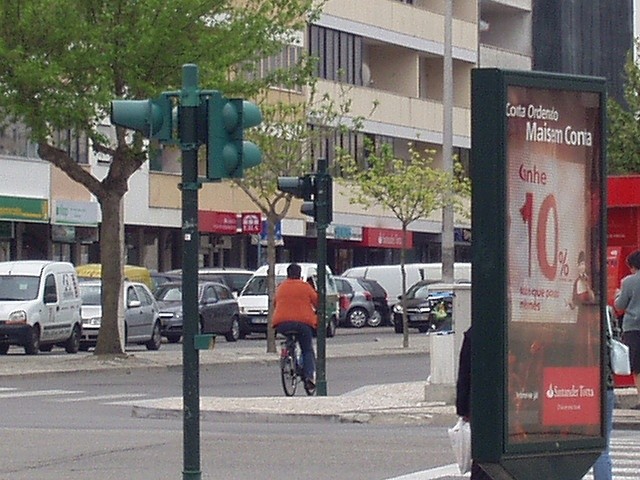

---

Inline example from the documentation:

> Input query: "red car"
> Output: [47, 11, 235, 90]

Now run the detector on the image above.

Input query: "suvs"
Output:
[329, 275, 373, 329]
[358, 278, 390, 327]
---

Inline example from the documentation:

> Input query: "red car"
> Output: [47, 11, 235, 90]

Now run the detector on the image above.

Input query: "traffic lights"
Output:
[275, 174, 302, 200]
[208, 89, 263, 185]
[299, 201, 317, 221]
[108, 92, 173, 150]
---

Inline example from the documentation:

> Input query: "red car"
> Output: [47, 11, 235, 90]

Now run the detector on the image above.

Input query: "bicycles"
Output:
[269, 330, 319, 398]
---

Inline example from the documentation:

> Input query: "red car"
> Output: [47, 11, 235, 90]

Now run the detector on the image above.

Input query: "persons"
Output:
[592, 304, 614, 480]
[271, 264, 318, 390]
[456, 326, 488, 479]
[612, 250, 640, 411]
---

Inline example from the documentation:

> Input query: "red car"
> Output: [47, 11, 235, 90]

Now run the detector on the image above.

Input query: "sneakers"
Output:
[304, 380, 315, 389]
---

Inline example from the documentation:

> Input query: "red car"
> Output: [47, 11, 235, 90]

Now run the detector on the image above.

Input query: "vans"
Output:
[239, 258, 337, 338]
[71, 260, 152, 300]
[165, 266, 253, 296]
[1, 259, 82, 355]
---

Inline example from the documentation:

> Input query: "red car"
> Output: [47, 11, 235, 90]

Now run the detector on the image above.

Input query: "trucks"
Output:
[345, 258, 471, 327]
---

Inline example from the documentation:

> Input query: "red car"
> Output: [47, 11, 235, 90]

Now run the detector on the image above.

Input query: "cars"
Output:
[156, 284, 239, 341]
[393, 276, 467, 336]
[77, 282, 160, 348]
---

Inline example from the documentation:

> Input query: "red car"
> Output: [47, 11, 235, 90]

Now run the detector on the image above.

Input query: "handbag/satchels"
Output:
[447, 422, 473, 475]
[610, 339, 631, 376]
